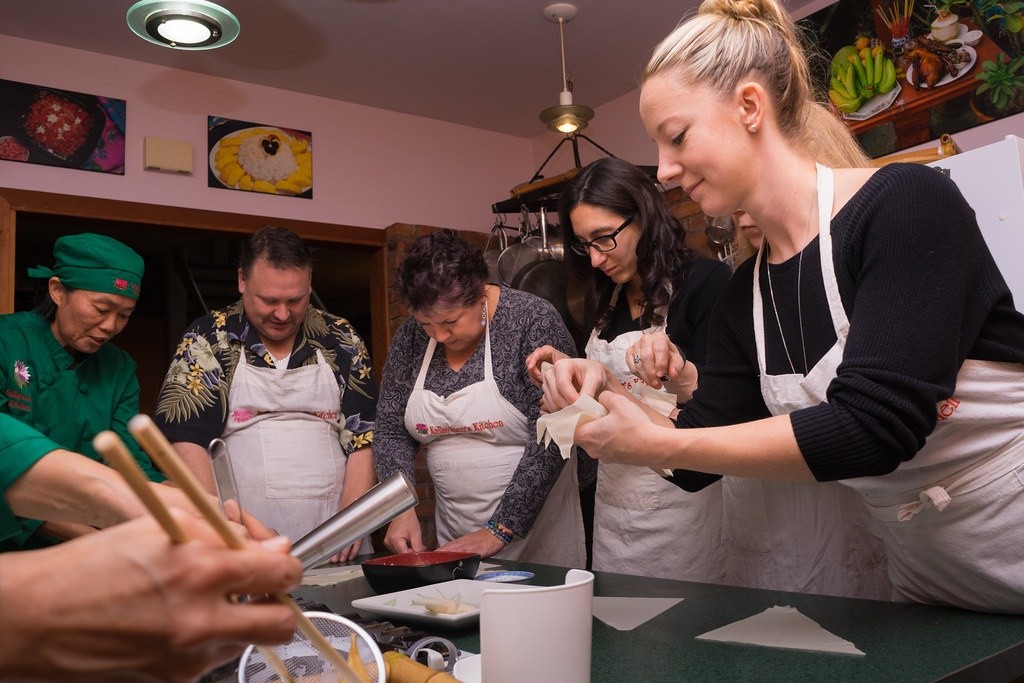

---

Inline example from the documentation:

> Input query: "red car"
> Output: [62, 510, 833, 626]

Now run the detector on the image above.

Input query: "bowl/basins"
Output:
[361, 551, 481, 595]
[960, 30, 983, 47]
[475, 570, 535, 586]
[947, 48, 969, 70]
[944, 39, 964, 49]
[931, 14, 959, 39]
[17, 88, 105, 169]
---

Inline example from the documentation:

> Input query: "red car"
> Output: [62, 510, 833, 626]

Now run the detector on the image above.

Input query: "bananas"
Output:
[828, 47, 896, 112]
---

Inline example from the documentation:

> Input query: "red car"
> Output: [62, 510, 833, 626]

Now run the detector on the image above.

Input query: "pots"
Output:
[511, 206, 571, 316]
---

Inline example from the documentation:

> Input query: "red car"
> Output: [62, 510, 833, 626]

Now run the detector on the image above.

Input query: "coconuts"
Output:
[930, 14, 959, 42]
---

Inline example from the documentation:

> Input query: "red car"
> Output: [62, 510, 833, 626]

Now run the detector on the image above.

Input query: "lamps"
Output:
[126, 0, 239, 51]
[538, 4, 596, 134]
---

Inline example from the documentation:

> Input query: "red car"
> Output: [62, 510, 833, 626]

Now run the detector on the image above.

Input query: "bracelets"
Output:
[484, 521, 514, 545]
[657, 344, 686, 380]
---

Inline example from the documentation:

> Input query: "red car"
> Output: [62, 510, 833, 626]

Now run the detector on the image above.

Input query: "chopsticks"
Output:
[93, 413, 365, 683]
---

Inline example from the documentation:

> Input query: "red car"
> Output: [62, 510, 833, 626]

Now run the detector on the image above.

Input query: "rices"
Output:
[239, 134, 296, 182]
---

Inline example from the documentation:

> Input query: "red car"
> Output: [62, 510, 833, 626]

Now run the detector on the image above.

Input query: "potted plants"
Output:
[968, 52, 1024, 124]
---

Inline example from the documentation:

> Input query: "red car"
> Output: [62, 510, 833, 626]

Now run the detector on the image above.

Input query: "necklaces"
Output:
[766, 191, 809, 377]
[275, 358, 287, 363]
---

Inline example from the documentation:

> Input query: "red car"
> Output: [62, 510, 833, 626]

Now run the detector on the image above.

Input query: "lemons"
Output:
[856, 36, 886, 66]
[215, 128, 312, 195]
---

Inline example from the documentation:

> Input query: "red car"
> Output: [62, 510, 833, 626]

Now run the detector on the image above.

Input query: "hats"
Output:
[26, 233, 144, 301]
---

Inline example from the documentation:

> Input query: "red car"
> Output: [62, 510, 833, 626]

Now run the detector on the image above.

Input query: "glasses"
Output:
[570, 213, 636, 256]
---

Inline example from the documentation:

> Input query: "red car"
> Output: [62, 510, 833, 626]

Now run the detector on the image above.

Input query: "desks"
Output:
[190, 547, 1024, 683]
[817, 18, 1011, 159]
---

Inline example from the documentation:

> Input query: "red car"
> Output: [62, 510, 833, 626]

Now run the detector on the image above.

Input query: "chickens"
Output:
[902, 37, 972, 90]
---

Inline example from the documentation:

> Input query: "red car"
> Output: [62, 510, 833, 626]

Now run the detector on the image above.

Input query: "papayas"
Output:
[831, 46, 858, 77]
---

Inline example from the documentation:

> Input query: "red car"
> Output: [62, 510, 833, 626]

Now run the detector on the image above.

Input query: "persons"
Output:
[153, 224, 379, 566]
[0, 234, 185, 553]
[541, 0, 1024, 615]
[526, 156, 735, 587]
[371, 229, 587, 570]
[0, 413, 304, 683]
[625, 204, 891, 600]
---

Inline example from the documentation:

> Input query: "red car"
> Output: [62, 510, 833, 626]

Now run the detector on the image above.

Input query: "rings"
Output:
[633, 355, 641, 363]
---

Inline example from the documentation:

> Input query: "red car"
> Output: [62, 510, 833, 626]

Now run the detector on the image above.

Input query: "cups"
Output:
[892, 37, 906, 49]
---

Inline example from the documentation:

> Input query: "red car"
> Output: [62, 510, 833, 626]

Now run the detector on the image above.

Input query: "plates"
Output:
[842, 81, 902, 121]
[453, 653, 483, 683]
[906, 45, 977, 88]
[209, 126, 313, 196]
[0, 135, 30, 162]
[926, 23, 968, 42]
[892, 47, 904, 54]
[351, 579, 545, 627]
